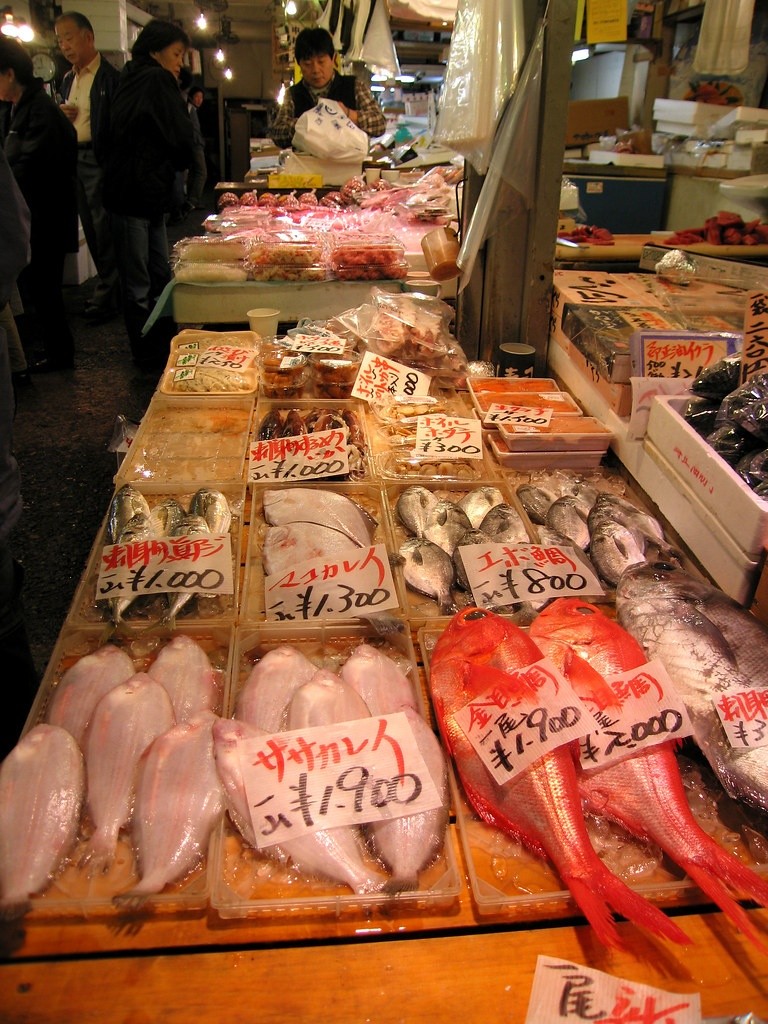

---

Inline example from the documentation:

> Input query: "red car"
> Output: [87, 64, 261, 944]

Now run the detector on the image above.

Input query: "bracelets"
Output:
[347, 107, 350, 118]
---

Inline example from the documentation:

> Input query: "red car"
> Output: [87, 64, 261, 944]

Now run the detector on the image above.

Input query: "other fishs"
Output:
[0, 480, 768, 954]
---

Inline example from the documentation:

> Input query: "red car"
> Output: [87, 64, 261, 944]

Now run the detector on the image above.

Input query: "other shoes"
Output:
[33, 357, 74, 372]
[84, 305, 110, 317]
[87, 298, 97, 305]
[196, 203, 205, 210]
[16, 371, 33, 390]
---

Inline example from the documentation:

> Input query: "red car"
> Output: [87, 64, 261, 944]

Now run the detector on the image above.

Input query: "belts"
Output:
[78, 142, 92, 150]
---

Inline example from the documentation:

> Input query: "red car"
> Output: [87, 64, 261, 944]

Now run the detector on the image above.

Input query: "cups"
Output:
[496, 343, 537, 378]
[246, 308, 280, 338]
[405, 280, 442, 298]
[365, 168, 381, 185]
[421, 226, 461, 282]
[381, 170, 400, 182]
[407, 270, 430, 280]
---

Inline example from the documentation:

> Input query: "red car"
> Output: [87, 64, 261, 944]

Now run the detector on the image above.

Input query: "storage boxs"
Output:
[243, 232, 411, 281]
[555, 96, 767, 622]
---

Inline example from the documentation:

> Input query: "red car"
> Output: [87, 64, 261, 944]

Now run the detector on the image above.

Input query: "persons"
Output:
[0, 9, 209, 761]
[269, 27, 386, 157]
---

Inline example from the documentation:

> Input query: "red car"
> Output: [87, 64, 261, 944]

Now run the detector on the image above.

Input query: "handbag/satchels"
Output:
[292, 98, 368, 164]
